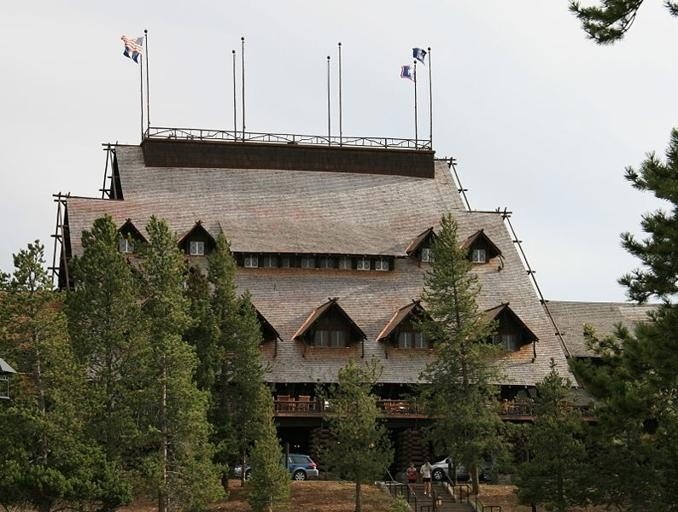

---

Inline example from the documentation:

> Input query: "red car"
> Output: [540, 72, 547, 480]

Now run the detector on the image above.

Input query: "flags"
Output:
[120, 34, 145, 54]
[411, 47, 427, 63]
[399, 64, 415, 82]
[122, 46, 139, 64]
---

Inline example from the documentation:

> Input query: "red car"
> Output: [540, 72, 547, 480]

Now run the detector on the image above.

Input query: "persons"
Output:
[406, 462, 417, 494]
[419, 457, 434, 497]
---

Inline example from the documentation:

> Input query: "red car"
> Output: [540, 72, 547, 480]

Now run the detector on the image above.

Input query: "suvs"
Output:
[241, 451, 321, 482]
[427, 453, 490, 482]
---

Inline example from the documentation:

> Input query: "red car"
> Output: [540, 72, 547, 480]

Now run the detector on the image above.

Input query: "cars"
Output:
[232, 463, 245, 479]
